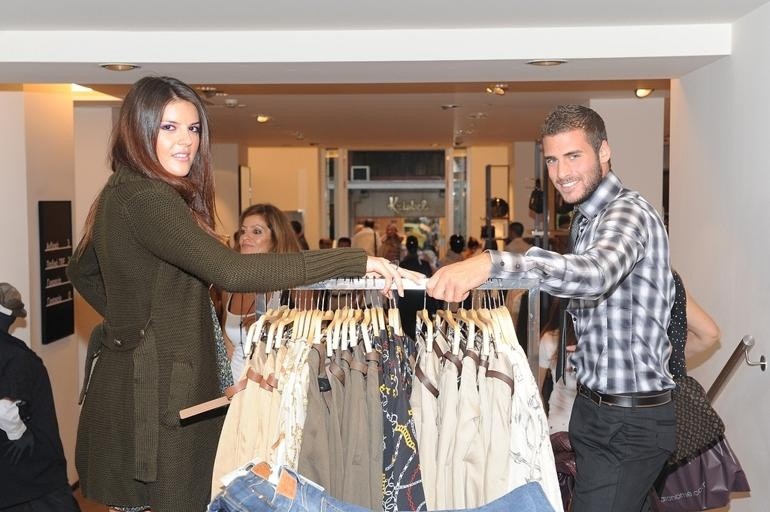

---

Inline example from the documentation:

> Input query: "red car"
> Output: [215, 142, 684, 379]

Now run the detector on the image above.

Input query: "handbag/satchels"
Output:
[665, 375, 727, 469]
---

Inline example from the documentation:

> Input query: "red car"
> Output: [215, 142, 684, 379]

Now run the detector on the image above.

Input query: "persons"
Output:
[315, 218, 530, 340]
[65, 77, 427, 512]
[218, 204, 309, 385]
[0, 283, 81, 512]
[427, 105, 722, 512]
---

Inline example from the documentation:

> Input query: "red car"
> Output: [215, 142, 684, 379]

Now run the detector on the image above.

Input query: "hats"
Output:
[405, 236, 419, 247]
[450, 234, 464, 245]
[0, 281, 28, 320]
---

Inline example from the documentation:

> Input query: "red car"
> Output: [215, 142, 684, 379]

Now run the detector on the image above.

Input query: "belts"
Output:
[576, 380, 672, 409]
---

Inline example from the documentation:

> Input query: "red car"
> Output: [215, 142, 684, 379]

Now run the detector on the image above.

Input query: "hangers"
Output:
[235, 277, 521, 359]
